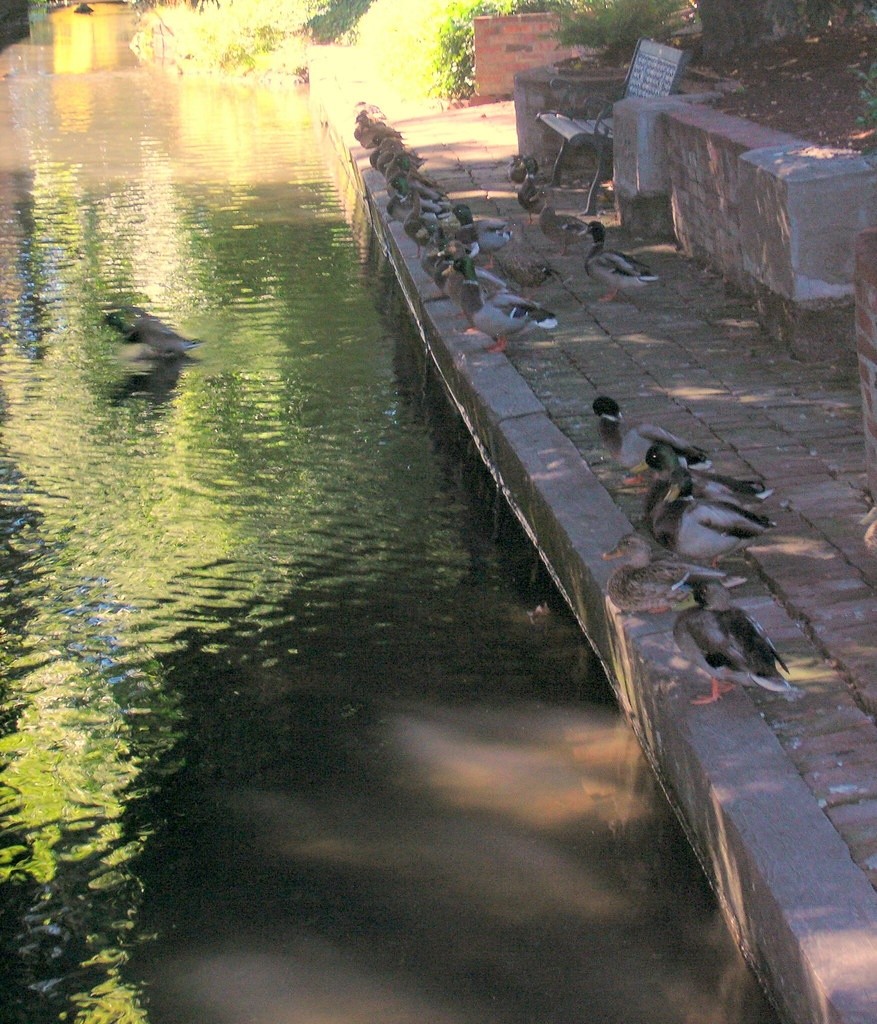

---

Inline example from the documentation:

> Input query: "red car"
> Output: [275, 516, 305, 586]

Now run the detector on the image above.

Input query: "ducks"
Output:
[647, 466, 779, 569]
[89, 307, 207, 361]
[629, 442, 775, 520]
[593, 396, 713, 494]
[672, 579, 800, 704]
[441, 256, 558, 354]
[494, 223, 558, 300]
[576, 221, 660, 303]
[601, 534, 748, 615]
[353, 101, 513, 336]
[505, 154, 547, 226]
[529, 186, 592, 257]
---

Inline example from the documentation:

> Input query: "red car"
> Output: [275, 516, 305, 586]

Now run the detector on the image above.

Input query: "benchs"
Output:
[538, 35, 689, 215]
